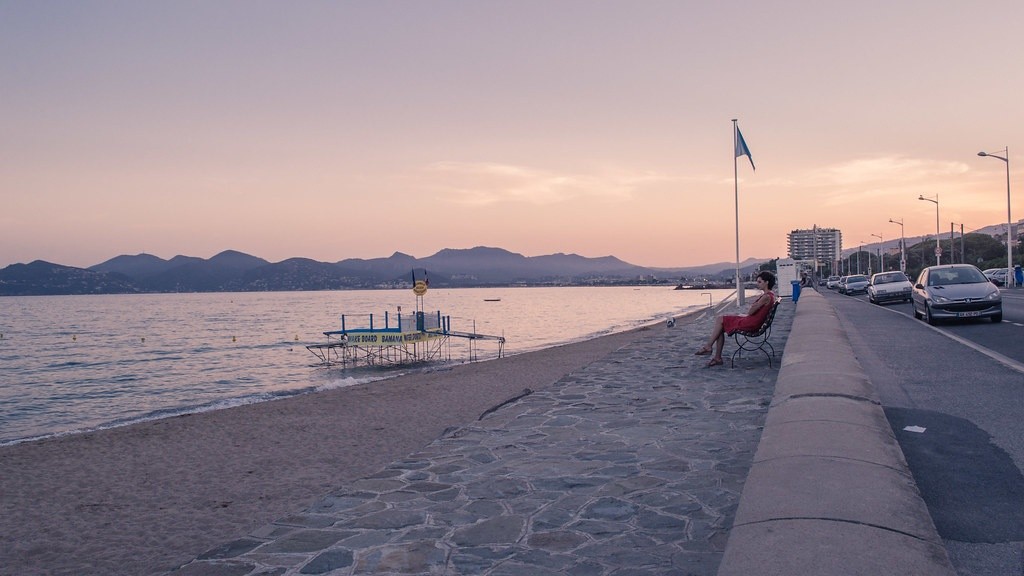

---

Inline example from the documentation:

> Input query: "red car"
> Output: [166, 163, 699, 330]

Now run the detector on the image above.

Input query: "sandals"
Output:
[707, 357, 724, 367]
[694, 347, 712, 355]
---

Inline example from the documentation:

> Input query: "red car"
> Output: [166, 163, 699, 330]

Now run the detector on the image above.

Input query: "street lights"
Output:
[978, 146, 1016, 287]
[918, 193, 942, 266]
[861, 241, 874, 275]
[889, 217, 905, 273]
[871, 232, 883, 273]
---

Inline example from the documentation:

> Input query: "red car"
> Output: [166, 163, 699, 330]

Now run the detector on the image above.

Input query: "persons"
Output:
[694, 272, 775, 366]
[799, 273, 808, 287]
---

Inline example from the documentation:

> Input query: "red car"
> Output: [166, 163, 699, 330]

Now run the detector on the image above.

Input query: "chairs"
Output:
[727, 297, 781, 367]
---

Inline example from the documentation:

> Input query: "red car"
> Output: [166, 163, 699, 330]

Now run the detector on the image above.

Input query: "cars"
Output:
[826, 274, 872, 295]
[982, 267, 1024, 288]
[911, 264, 1002, 326]
[866, 271, 914, 305]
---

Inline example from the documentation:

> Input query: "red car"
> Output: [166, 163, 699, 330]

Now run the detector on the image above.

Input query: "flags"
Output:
[412, 268, 416, 287]
[425, 269, 431, 287]
[735, 125, 755, 171]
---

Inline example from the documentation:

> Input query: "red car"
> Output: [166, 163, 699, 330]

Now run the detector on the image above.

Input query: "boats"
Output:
[483, 299, 501, 302]
